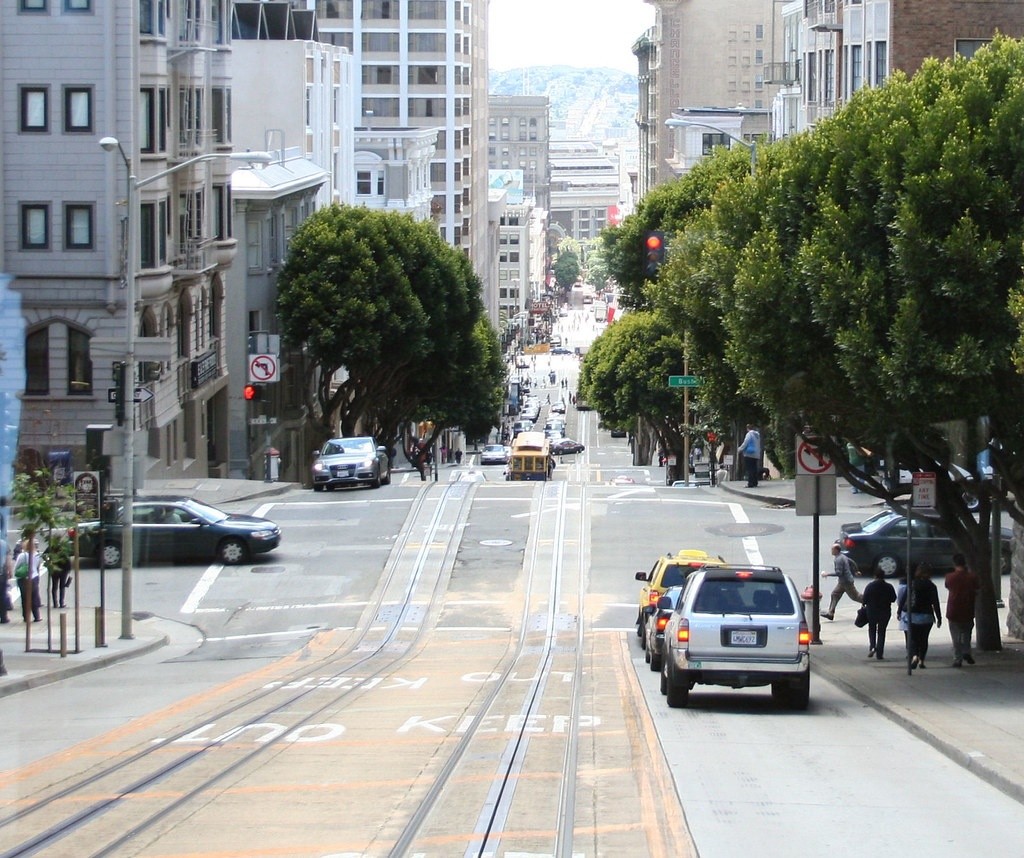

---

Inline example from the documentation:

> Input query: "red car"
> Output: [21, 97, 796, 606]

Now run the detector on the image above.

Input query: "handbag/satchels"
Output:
[64, 576, 73, 588]
[855, 603, 868, 628]
[903, 580, 917, 612]
[744, 432, 756, 454]
[14, 552, 29, 578]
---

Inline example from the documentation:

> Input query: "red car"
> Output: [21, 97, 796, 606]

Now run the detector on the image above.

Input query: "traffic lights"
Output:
[244, 384, 263, 402]
[140, 360, 161, 383]
[643, 230, 665, 275]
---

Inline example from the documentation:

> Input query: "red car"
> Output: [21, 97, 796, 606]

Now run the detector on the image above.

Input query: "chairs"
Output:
[753, 589, 774, 611]
[706, 590, 731, 610]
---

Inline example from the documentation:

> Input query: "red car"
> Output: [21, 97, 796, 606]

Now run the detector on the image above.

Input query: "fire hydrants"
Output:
[269, 448, 281, 482]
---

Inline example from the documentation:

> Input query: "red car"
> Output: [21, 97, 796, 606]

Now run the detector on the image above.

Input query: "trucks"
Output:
[593, 301, 607, 322]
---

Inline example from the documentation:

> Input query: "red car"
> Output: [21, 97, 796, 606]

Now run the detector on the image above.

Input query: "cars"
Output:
[504, 397, 585, 464]
[67, 495, 282, 570]
[480, 445, 508, 465]
[672, 481, 710, 488]
[835, 461, 1013, 579]
[312, 436, 391, 492]
[550, 294, 593, 355]
[642, 585, 747, 672]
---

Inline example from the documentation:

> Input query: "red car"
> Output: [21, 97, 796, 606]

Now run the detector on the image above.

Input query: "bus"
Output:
[576, 391, 594, 411]
[506, 432, 551, 481]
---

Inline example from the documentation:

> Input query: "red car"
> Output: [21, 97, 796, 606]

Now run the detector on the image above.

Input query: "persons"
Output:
[945, 552, 977, 668]
[558, 312, 595, 332]
[161, 507, 191, 524]
[455, 448, 462, 464]
[846, 438, 873, 494]
[738, 424, 760, 487]
[689, 448, 702, 463]
[658, 438, 665, 467]
[895, 563, 942, 669]
[565, 337, 568, 345]
[523, 369, 567, 388]
[716, 464, 728, 487]
[528, 353, 536, 365]
[545, 393, 551, 404]
[0, 529, 43, 622]
[440, 445, 453, 464]
[862, 566, 897, 660]
[561, 390, 575, 408]
[43, 535, 72, 608]
[820, 545, 868, 621]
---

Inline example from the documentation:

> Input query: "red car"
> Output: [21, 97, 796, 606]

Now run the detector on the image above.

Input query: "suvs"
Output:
[657, 563, 811, 708]
[635, 550, 727, 650]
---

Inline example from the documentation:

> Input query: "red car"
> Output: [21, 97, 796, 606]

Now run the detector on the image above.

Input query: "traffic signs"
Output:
[108, 387, 155, 404]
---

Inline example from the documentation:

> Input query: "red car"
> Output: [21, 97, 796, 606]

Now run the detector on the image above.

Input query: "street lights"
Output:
[664, 119, 756, 177]
[100, 136, 274, 642]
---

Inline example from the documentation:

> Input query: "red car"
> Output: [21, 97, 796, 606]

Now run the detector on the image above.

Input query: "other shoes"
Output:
[963, 653, 975, 664]
[919, 663, 926, 669]
[869, 648, 875, 657]
[953, 659, 961, 667]
[911, 658, 919, 669]
[820, 613, 834, 620]
[878, 655, 884, 660]
